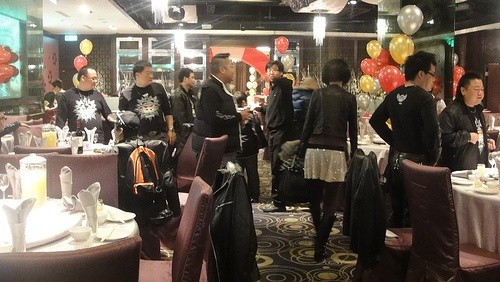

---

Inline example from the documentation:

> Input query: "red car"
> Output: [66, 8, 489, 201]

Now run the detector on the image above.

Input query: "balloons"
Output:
[432, 54, 465, 96]
[356, 4, 423, 112]
[276, 35, 295, 71]
[72, 39, 93, 88]
[0, 44, 19, 83]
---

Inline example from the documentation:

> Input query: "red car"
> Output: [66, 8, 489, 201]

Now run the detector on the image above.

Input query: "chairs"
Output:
[0, 109, 261, 282]
[342, 113, 500, 282]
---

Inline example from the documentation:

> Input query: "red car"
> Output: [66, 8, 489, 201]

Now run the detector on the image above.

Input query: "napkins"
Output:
[4, 198, 36, 250]
[78, 182, 101, 231]
[20, 131, 31, 146]
[63, 195, 84, 212]
[5, 163, 21, 198]
[59, 166, 72, 198]
[70, 137, 78, 154]
[489, 115, 495, 130]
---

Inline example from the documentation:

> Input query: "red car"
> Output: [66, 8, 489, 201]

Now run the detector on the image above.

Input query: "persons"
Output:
[108, 51, 254, 219]
[55, 66, 115, 145]
[368, 52, 439, 228]
[234, 61, 294, 213]
[428, 87, 447, 124]
[435, 72, 490, 172]
[0, 105, 31, 149]
[40, 78, 66, 111]
[297, 59, 358, 262]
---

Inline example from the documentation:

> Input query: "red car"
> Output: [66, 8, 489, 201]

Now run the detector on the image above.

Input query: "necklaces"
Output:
[470, 107, 476, 113]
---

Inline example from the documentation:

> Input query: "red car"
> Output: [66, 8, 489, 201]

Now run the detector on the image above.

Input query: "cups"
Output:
[19, 131, 32, 147]
[2, 135, 14, 155]
[61, 183, 73, 198]
[71, 135, 83, 154]
[98, 199, 104, 206]
[477, 164, 485, 174]
[11, 223, 26, 251]
[85, 204, 98, 233]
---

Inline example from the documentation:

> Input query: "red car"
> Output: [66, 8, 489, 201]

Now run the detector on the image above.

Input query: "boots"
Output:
[313, 210, 337, 260]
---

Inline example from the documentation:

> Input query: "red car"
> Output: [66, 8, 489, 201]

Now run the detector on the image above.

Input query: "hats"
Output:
[106, 111, 139, 130]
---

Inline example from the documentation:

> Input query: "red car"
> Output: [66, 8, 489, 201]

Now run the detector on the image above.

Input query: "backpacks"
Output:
[127, 147, 161, 196]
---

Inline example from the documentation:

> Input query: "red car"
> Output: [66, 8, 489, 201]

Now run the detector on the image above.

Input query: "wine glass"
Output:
[0, 173, 9, 200]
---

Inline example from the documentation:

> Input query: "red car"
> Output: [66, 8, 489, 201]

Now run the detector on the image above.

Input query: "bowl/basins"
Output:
[69, 226, 92, 241]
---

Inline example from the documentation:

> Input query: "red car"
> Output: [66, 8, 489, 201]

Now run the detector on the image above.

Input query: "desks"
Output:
[451, 168, 500, 253]
[0, 198, 140, 253]
[357, 142, 390, 176]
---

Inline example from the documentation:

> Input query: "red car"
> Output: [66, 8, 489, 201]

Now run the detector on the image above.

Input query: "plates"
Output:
[453, 178, 472, 185]
[475, 187, 499, 193]
[106, 212, 136, 221]
[95, 227, 133, 240]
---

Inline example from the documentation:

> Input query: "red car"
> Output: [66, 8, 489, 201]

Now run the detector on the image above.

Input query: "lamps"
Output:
[311, 9, 329, 47]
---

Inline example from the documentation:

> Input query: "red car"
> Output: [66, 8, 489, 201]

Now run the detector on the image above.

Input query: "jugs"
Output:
[19, 153, 47, 200]
[41, 123, 57, 148]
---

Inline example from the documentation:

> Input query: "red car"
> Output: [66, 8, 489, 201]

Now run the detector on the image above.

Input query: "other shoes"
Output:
[263, 203, 286, 213]
